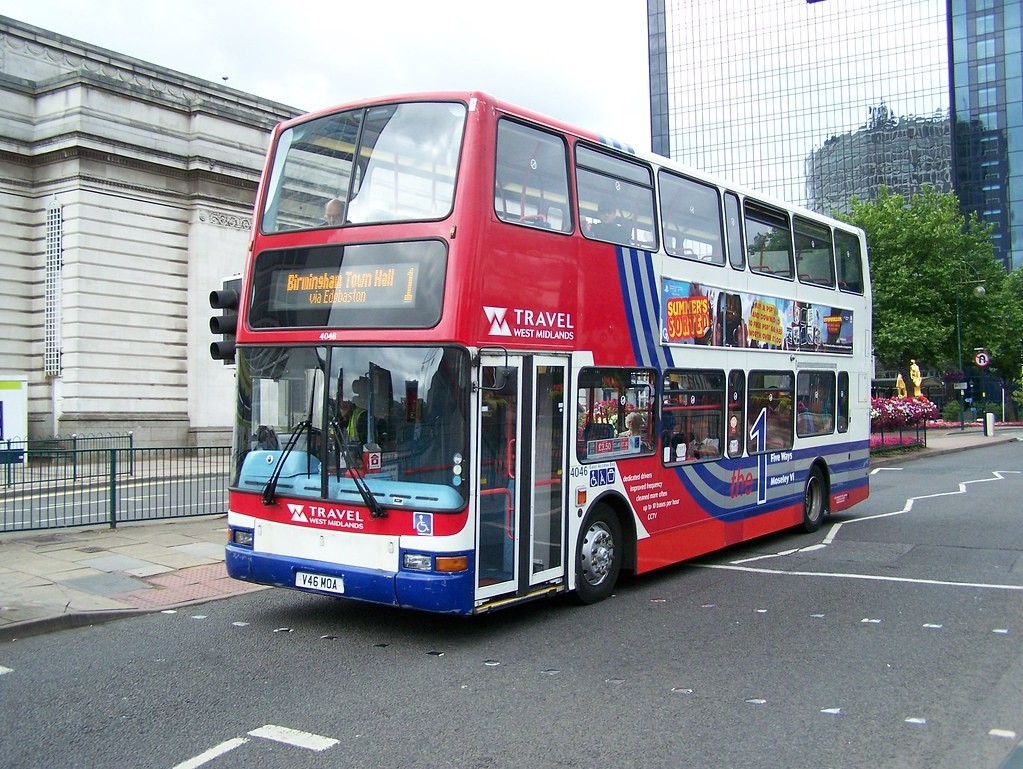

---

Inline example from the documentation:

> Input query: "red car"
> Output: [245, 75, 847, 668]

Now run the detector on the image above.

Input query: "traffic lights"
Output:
[209, 288, 237, 360]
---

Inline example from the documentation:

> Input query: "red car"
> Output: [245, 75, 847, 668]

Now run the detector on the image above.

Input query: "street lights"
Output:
[952, 261, 986, 429]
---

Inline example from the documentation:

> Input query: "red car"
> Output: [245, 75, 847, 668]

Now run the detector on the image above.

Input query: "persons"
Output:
[619, 411, 647, 444]
[685, 419, 719, 458]
[588, 199, 630, 244]
[910, 359, 924, 396]
[895, 373, 908, 398]
[328, 383, 384, 454]
[320, 198, 353, 225]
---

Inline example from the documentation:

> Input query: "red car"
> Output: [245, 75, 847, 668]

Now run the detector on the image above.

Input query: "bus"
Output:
[224, 91, 874, 616]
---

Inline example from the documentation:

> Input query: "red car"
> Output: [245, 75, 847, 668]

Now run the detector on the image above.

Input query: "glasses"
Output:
[325, 213, 342, 219]
